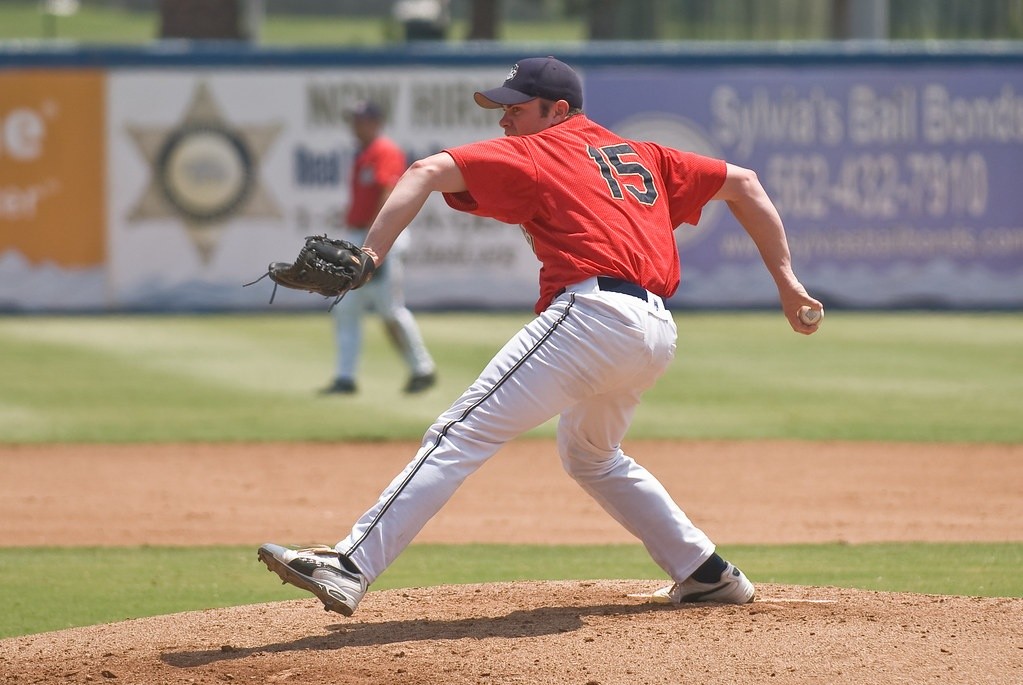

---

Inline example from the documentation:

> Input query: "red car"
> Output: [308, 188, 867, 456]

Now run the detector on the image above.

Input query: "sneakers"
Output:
[651, 561, 755, 605]
[257, 543, 368, 617]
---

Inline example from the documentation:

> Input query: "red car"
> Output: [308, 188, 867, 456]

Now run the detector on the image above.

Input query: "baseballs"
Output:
[798, 306, 823, 326]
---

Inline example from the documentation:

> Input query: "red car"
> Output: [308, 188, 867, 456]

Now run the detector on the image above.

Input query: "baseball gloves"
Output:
[241, 233, 378, 312]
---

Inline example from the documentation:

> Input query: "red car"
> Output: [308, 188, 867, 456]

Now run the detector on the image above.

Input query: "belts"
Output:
[554, 276, 666, 309]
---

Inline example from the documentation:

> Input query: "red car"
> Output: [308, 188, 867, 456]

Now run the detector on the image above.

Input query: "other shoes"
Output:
[407, 374, 433, 393]
[322, 379, 356, 393]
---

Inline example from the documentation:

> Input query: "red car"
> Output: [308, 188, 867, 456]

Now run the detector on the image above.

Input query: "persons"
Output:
[259, 56, 822, 618]
[319, 100, 438, 396]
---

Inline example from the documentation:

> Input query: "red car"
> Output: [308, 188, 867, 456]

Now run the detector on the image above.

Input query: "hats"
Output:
[348, 104, 380, 120]
[474, 56, 583, 109]
